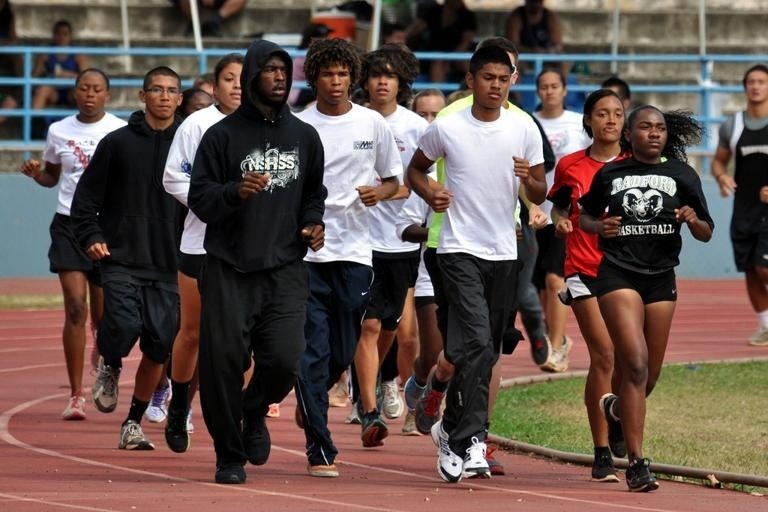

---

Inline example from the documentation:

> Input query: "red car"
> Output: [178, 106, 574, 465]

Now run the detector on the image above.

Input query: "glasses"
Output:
[146, 87, 179, 97]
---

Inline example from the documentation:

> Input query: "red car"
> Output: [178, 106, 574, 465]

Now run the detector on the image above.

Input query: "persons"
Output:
[18, 37, 629, 483]
[578, 104, 716, 493]
[710, 63, 768, 346]
[3, 0, 567, 144]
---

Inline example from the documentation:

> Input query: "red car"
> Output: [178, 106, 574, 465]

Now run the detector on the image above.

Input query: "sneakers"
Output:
[328, 364, 504, 482]
[532, 329, 570, 373]
[215, 402, 339, 482]
[61, 356, 194, 453]
[591, 392, 659, 493]
[749, 326, 768, 346]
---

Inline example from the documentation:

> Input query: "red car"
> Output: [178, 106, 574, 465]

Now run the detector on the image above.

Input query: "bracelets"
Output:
[715, 171, 728, 182]
